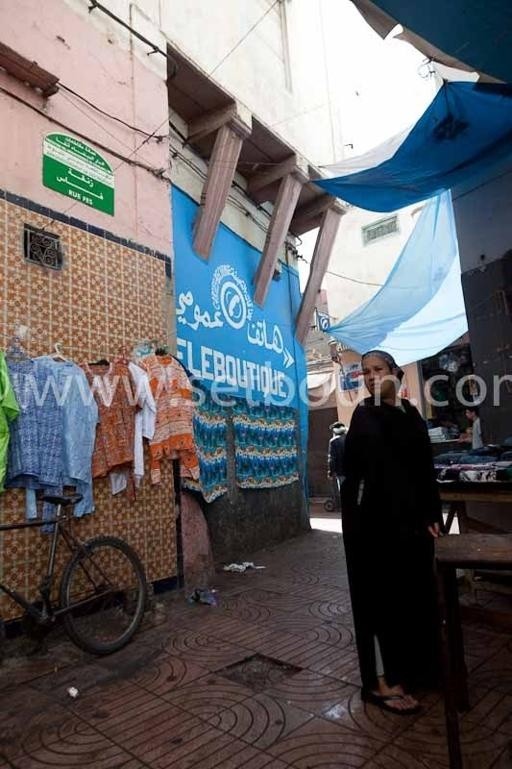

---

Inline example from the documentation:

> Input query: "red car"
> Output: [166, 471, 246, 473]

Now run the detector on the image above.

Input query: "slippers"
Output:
[360, 685, 419, 714]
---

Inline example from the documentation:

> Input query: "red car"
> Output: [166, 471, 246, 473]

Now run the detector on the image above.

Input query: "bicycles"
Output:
[0, 493, 148, 656]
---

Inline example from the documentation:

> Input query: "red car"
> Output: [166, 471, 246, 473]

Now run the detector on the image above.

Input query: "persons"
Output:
[339, 349, 445, 714]
[457, 404, 483, 452]
[325, 422, 347, 495]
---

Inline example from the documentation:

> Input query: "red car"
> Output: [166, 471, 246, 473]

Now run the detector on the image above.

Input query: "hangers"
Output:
[3, 337, 170, 373]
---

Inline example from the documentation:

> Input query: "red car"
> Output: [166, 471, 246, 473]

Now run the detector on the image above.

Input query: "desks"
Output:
[432, 534, 512, 769]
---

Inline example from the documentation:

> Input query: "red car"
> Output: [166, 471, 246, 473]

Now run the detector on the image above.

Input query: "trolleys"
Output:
[307, 492, 337, 513]
